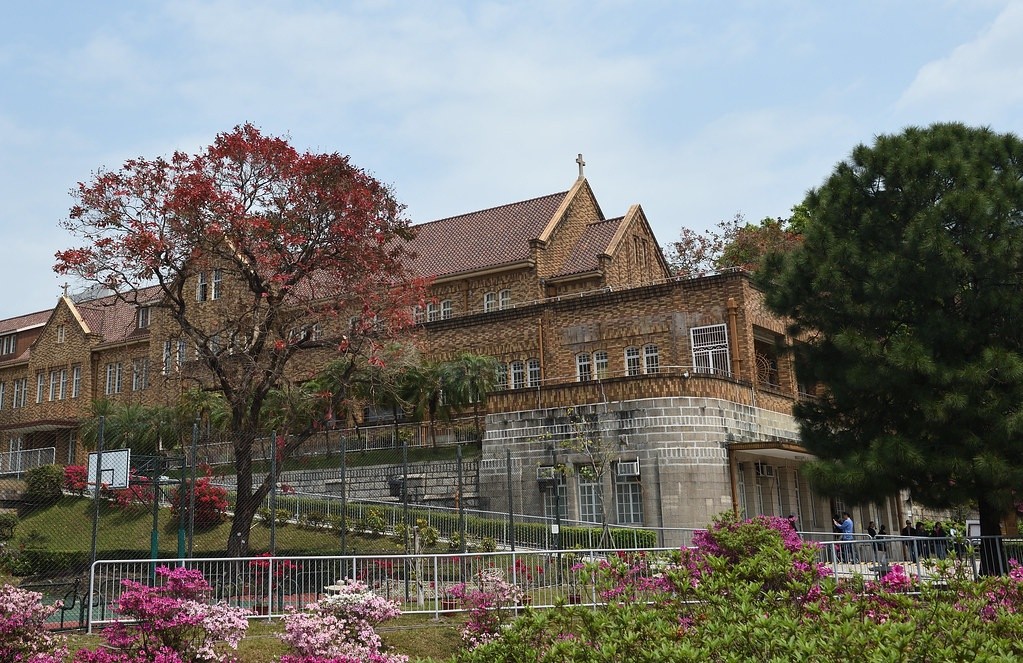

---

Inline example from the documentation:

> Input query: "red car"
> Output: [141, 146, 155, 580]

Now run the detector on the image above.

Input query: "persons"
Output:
[788, 511, 965, 562]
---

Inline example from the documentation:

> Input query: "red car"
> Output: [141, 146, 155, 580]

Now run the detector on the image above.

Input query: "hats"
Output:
[906, 520, 912, 524]
